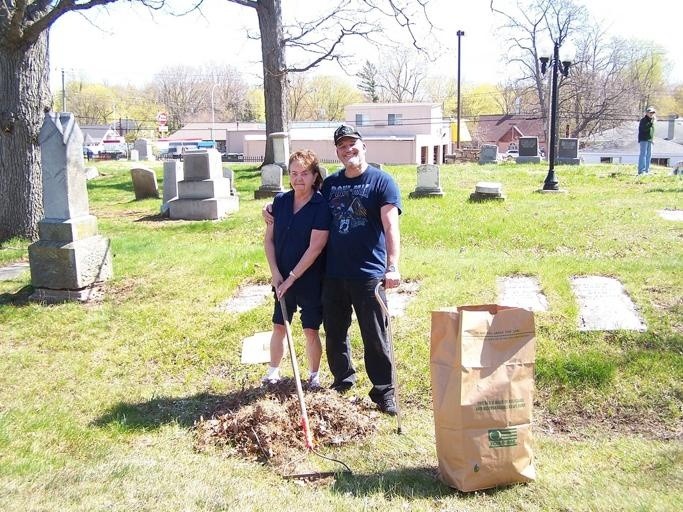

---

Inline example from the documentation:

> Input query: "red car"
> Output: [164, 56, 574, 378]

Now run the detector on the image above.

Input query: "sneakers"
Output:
[376, 400, 398, 415]
[329, 382, 351, 395]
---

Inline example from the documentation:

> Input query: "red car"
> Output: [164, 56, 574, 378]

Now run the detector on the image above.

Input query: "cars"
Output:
[538, 149, 545, 157]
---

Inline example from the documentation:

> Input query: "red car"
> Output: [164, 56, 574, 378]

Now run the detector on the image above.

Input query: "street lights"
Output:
[452, 30, 466, 151]
[210, 83, 224, 149]
[532, 39, 576, 193]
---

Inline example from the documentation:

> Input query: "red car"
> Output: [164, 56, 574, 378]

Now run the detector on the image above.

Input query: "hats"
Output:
[646, 106, 656, 114]
[334, 125, 360, 142]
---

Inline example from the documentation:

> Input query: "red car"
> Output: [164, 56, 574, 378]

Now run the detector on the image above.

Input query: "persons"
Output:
[261, 125, 403, 413]
[638, 106, 656, 176]
[259, 150, 331, 387]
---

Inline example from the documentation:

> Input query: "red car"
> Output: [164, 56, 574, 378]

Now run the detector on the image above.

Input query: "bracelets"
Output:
[288, 270, 297, 279]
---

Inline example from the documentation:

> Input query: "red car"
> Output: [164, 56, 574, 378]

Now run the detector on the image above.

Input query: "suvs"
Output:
[505, 150, 518, 157]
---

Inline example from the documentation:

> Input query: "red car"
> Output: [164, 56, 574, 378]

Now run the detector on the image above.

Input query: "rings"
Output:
[278, 290, 281, 293]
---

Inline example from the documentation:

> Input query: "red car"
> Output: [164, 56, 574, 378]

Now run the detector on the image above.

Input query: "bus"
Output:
[168, 142, 199, 157]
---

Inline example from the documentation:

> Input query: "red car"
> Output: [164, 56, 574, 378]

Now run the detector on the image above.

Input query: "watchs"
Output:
[385, 265, 400, 272]
